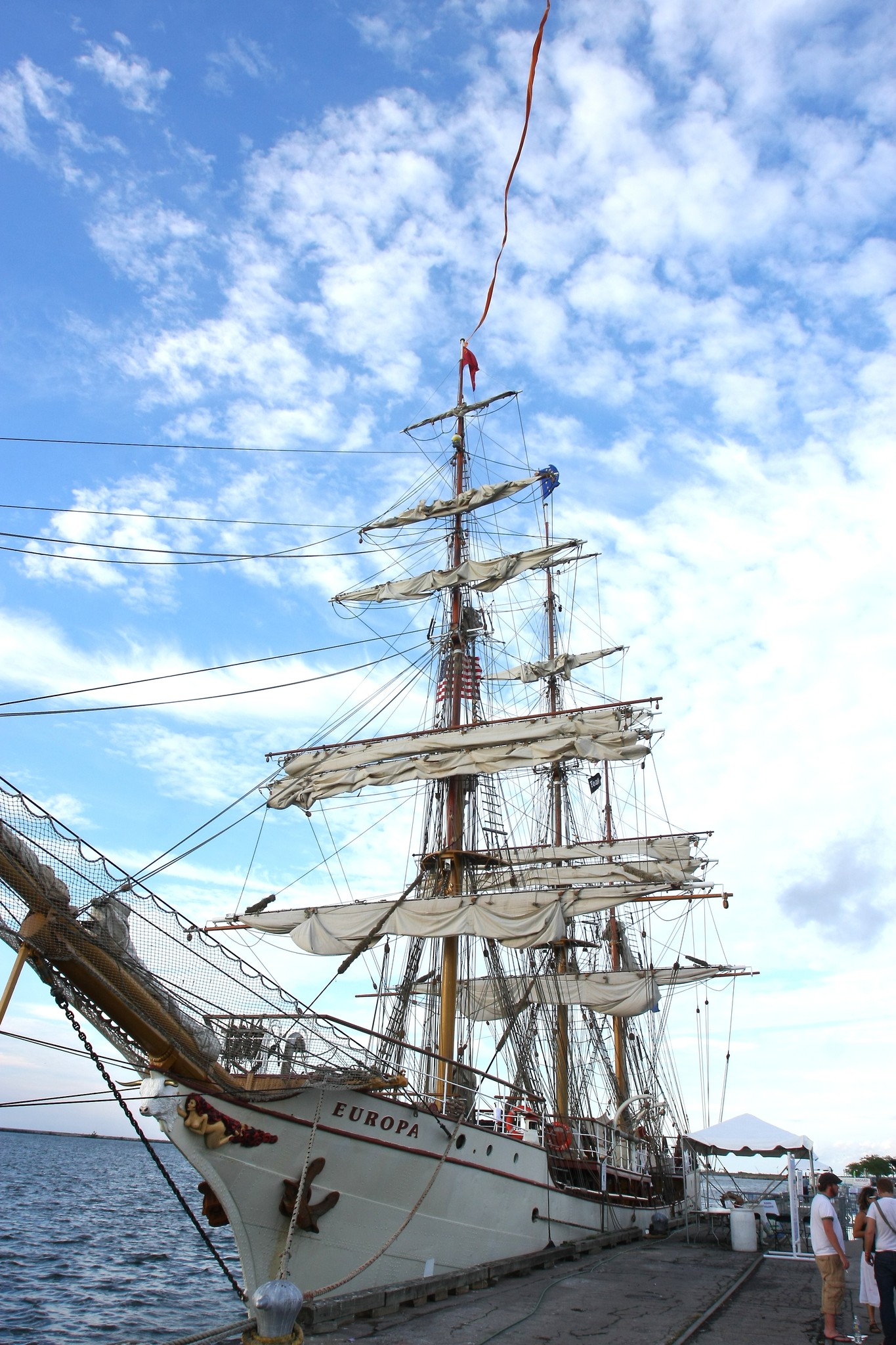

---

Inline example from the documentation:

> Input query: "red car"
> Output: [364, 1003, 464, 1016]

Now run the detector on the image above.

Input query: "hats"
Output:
[818, 1172, 842, 1184]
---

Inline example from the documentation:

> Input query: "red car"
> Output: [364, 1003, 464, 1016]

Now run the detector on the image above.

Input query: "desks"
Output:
[691, 1210, 730, 1246]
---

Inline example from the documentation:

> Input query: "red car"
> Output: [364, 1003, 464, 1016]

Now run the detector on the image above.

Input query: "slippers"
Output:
[819, 1311, 843, 1316]
[869, 1324, 880, 1333]
[823, 1332, 851, 1342]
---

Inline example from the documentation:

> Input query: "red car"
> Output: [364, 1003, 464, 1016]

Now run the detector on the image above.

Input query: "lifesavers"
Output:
[546, 1122, 573, 1150]
[506, 1106, 540, 1141]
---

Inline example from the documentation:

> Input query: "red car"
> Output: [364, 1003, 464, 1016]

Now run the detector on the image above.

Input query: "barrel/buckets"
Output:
[730, 1208, 757, 1252]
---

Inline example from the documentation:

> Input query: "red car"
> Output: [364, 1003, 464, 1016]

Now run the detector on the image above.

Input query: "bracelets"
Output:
[865, 1252, 870, 1254]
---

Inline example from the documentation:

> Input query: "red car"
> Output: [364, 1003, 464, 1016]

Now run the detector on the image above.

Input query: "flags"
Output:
[539, 464, 560, 501]
[462, 342, 480, 392]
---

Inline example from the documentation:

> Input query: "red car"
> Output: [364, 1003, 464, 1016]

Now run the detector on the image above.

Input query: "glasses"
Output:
[869, 1197, 880, 1200]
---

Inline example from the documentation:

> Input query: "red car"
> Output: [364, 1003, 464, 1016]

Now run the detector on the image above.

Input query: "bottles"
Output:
[853, 1316, 862, 1344]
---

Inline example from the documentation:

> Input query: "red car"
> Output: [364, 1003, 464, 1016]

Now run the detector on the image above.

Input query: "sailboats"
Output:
[2, 348, 768, 1320]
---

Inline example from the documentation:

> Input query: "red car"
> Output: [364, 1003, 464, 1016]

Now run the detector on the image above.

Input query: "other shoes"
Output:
[768, 1233, 775, 1237]
[760, 1242, 769, 1246]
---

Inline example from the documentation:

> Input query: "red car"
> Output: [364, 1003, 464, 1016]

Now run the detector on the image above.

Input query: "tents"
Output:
[680, 1114, 822, 1260]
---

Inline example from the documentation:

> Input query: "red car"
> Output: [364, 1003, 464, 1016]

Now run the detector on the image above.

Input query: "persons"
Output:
[177, 1092, 279, 1149]
[810, 1172, 853, 1342]
[853, 1177, 896, 1345]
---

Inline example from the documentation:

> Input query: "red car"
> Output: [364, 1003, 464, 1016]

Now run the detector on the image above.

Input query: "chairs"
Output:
[754, 1213, 812, 1253]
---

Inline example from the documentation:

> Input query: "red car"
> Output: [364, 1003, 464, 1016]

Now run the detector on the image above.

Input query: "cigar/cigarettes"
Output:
[846, 1270, 848, 1273]
